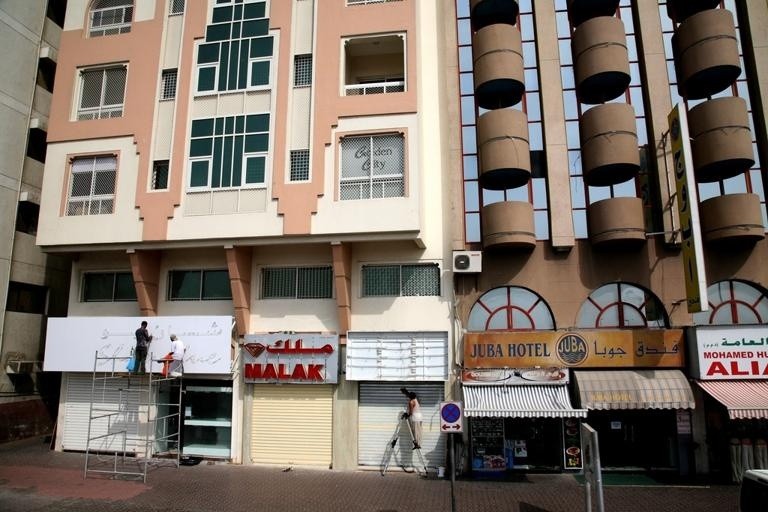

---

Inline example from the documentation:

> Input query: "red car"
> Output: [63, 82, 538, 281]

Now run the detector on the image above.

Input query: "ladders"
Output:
[382, 413, 428, 478]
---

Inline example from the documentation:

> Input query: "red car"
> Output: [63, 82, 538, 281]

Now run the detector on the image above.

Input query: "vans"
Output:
[737, 469, 768, 512]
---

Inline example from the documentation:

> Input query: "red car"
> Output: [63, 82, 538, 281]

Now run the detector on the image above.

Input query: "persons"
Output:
[400, 388, 421, 450]
[132, 321, 152, 376]
[157, 334, 186, 376]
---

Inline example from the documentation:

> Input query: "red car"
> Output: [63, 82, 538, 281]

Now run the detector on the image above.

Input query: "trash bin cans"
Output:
[671, 441, 696, 477]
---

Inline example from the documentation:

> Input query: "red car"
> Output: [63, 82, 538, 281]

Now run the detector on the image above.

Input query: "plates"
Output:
[566, 420, 578, 436]
[566, 447, 581, 455]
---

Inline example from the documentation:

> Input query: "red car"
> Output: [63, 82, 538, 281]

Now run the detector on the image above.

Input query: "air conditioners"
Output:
[453, 250, 483, 273]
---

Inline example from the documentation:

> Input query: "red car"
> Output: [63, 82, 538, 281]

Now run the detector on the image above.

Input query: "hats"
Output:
[170, 334, 178, 341]
